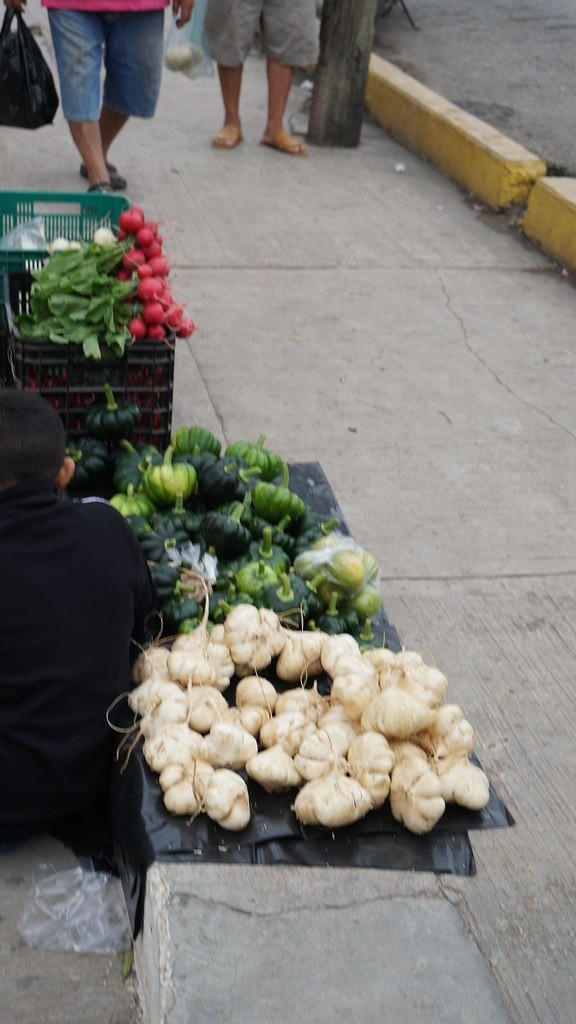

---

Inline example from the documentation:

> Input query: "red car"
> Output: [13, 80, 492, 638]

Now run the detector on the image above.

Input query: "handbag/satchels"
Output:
[0, 0, 59, 130]
[164, 0, 216, 80]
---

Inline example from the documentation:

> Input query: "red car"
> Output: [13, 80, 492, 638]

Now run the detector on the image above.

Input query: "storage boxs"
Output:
[1, 189, 128, 269]
[1, 269, 175, 458]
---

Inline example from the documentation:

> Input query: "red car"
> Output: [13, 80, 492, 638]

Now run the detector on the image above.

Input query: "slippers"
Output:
[260, 131, 308, 157]
[213, 126, 243, 148]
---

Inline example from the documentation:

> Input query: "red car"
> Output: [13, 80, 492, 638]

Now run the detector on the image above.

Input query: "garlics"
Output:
[130, 598, 491, 834]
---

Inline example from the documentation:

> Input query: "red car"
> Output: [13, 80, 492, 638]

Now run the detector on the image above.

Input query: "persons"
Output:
[0, 385, 167, 1024]
[5, 1, 324, 198]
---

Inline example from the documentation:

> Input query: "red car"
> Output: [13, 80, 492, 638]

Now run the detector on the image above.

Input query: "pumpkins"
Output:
[50, 383, 376, 632]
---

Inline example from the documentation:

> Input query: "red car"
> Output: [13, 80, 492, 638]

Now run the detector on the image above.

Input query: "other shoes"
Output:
[80, 162, 127, 189]
[88, 183, 114, 193]
